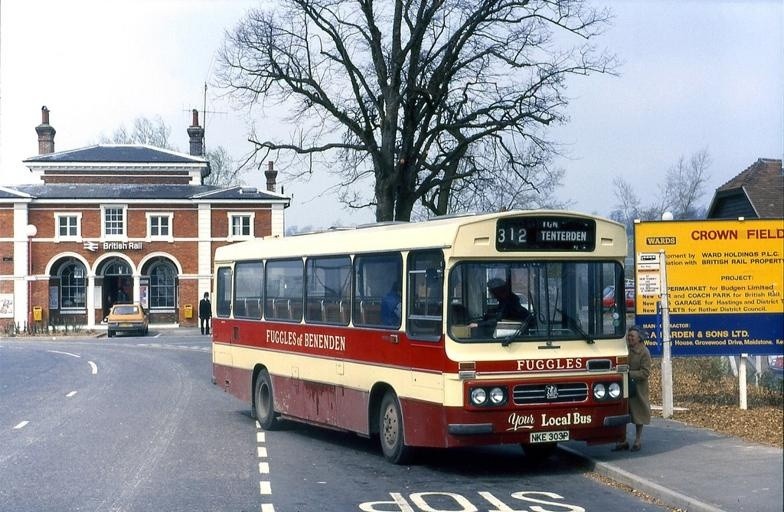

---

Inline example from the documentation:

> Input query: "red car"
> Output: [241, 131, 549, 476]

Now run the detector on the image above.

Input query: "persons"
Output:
[199, 292, 212, 335]
[116, 288, 126, 302]
[468, 277, 534, 335]
[612, 325, 652, 453]
[381, 282, 407, 326]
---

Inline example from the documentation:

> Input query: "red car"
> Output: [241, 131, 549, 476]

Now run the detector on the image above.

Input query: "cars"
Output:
[108, 303, 150, 337]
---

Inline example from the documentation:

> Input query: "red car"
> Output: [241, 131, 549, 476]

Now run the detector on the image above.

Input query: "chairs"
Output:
[229, 297, 384, 325]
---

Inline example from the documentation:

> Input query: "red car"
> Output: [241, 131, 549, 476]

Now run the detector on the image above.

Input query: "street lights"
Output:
[25, 225, 37, 334]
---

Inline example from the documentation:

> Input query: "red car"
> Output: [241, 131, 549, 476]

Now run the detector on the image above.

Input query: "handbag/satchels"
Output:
[629, 379, 636, 398]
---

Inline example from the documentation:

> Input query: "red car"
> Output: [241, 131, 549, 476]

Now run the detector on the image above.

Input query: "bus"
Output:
[212, 208, 631, 464]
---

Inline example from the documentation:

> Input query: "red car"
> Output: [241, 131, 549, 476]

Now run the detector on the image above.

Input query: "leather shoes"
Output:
[611, 443, 641, 452]
